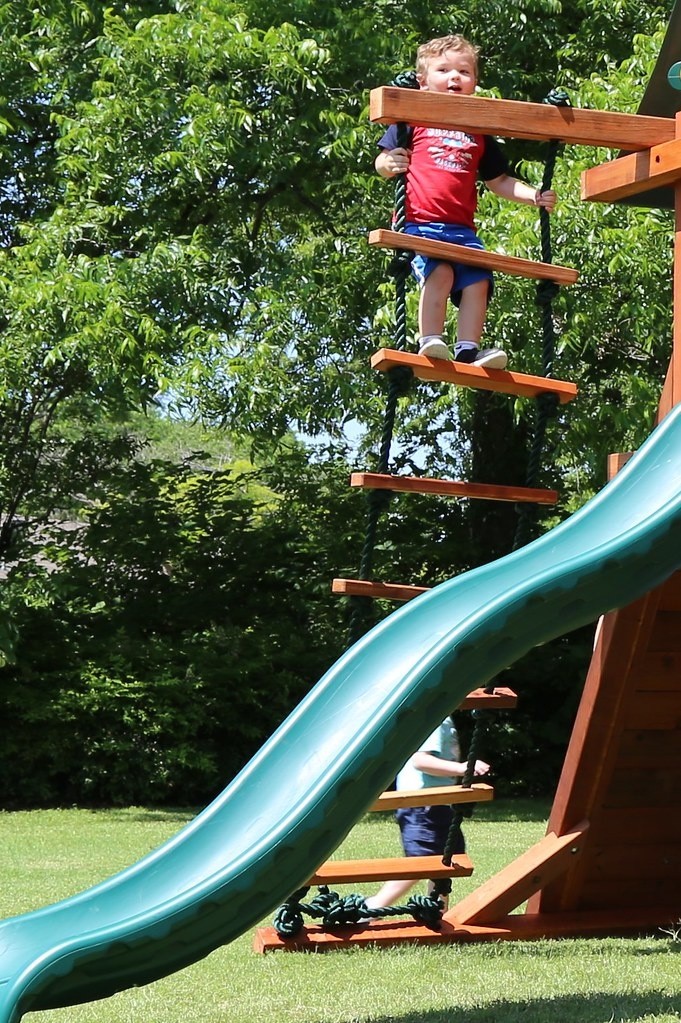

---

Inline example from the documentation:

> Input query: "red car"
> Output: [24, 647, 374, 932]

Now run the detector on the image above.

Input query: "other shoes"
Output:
[417, 338, 449, 382]
[454, 346, 508, 388]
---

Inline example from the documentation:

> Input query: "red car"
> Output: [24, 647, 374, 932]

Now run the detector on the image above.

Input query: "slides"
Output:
[1, 401, 681, 1023]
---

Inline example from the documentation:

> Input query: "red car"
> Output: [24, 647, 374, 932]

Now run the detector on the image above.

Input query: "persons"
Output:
[361, 718, 491, 909]
[376, 34, 560, 367]
